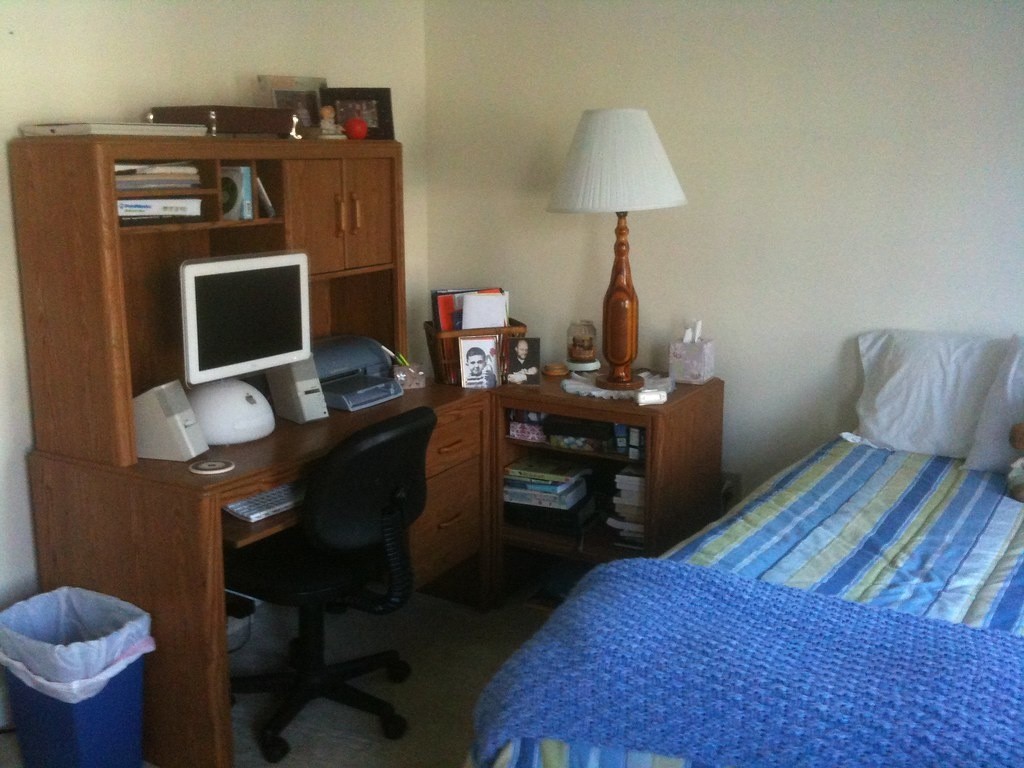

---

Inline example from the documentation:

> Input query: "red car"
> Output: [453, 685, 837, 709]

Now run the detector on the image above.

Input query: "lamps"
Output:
[547, 108, 687, 392]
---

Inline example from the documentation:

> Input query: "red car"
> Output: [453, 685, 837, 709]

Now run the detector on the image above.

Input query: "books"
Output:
[115, 160, 202, 221]
[429, 287, 510, 384]
[503, 460, 587, 510]
[607, 471, 647, 537]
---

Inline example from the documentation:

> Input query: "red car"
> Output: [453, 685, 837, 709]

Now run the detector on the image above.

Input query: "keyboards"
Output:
[222, 480, 311, 523]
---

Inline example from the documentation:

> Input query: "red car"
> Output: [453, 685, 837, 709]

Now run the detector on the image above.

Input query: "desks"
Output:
[24, 375, 497, 768]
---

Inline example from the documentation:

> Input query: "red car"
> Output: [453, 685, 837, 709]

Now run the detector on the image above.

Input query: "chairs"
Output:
[224, 405, 438, 764]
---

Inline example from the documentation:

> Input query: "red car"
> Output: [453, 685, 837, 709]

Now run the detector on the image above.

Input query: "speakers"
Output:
[267, 353, 329, 424]
[130, 380, 210, 461]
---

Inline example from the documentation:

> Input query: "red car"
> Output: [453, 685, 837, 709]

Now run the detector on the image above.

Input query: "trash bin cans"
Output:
[0, 586, 150, 768]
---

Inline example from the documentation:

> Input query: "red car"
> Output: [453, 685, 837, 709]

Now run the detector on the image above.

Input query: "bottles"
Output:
[566, 319, 596, 361]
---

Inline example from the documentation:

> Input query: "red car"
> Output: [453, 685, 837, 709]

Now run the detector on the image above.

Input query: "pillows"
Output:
[955, 334, 1024, 474]
[838, 328, 1012, 456]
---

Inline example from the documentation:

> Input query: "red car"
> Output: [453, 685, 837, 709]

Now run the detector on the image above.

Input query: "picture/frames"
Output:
[457, 335, 502, 389]
[320, 88, 395, 139]
[258, 73, 328, 139]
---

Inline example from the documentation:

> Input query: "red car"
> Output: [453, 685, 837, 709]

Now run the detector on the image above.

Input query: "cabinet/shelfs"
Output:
[491, 368, 724, 566]
[9, 137, 408, 468]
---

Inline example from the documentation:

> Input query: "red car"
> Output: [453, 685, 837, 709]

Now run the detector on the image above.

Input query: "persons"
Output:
[465, 347, 498, 389]
[506, 339, 538, 385]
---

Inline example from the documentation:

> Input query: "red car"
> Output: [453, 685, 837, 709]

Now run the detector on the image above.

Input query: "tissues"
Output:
[668, 319, 715, 385]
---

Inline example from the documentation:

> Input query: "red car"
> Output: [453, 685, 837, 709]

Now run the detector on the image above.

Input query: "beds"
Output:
[466, 431, 1024, 768]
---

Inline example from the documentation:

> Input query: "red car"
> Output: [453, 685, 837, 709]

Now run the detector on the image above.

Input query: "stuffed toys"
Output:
[1007, 423, 1024, 504]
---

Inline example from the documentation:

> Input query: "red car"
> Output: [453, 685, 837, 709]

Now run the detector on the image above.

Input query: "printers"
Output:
[310, 334, 405, 413]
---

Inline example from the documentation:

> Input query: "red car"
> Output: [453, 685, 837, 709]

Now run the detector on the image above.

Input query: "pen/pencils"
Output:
[381, 346, 410, 366]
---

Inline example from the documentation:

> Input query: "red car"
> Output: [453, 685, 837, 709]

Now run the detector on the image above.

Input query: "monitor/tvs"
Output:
[179, 250, 311, 446]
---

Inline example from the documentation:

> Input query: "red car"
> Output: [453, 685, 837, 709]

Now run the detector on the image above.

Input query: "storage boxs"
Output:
[146, 105, 293, 136]
[221, 165, 254, 221]
